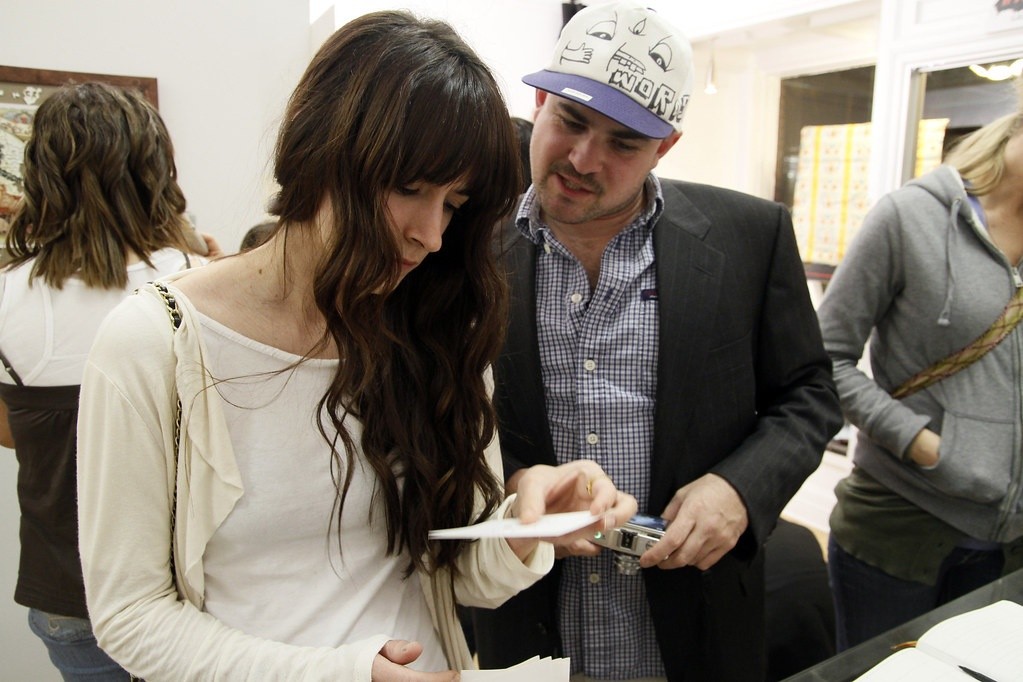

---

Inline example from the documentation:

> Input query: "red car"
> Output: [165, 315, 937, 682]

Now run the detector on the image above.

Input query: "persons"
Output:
[816, 109, 1023, 651]
[75, 10, 638, 681]
[1, 83, 218, 682]
[476, 0, 845, 681]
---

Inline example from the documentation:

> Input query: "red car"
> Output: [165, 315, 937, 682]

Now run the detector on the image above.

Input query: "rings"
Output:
[585, 475, 609, 495]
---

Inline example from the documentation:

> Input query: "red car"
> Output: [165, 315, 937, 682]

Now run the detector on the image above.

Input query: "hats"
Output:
[523, 0, 694, 140]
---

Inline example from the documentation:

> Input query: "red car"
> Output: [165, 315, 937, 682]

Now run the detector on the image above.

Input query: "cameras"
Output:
[590, 514, 670, 576]
